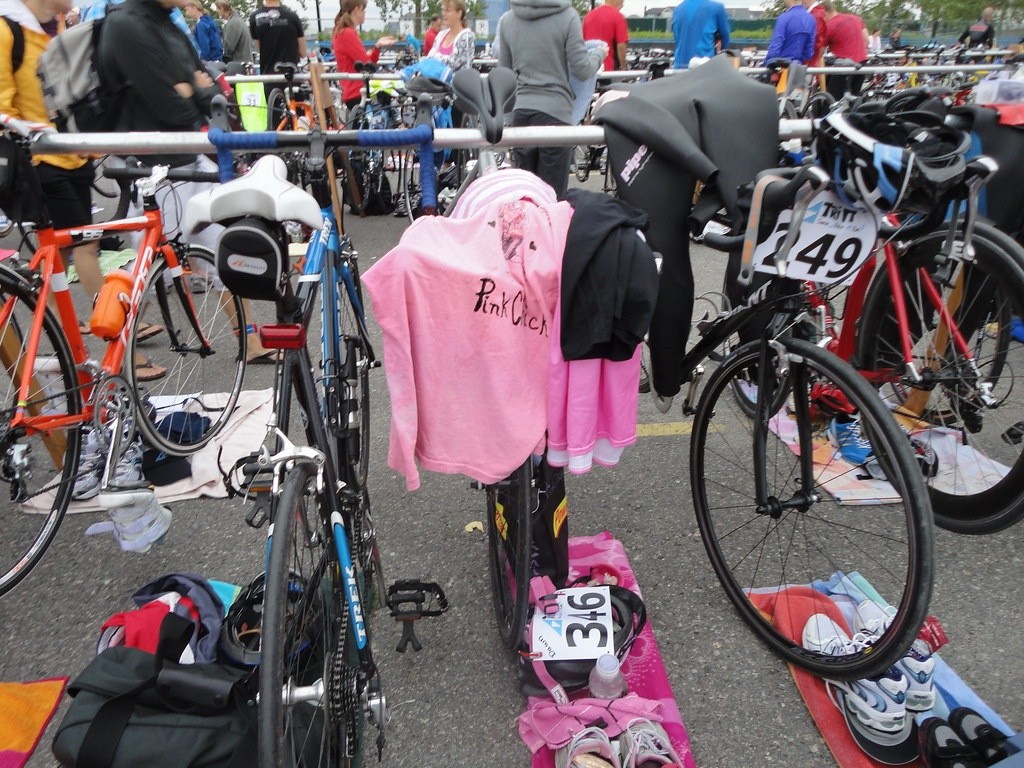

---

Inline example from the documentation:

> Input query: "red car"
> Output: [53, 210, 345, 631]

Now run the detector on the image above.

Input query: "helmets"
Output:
[809, 375, 856, 415]
[217, 565, 324, 673]
[810, 109, 974, 212]
[437, 163, 459, 191]
[517, 593, 635, 698]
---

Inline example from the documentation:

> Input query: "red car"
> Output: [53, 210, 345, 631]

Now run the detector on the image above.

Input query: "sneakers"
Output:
[878, 382, 955, 419]
[391, 191, 421, 217]
[618, 717, 684, 768]
[106, 400, 154, 489]
[555, 727, 621, 768]
[70, 398, 120, 500]
[827, 414, 874, 465]
[802, 613, 908, 732]
[918, 716, 986, 768]
[852, 599, 936, 711]
[947, 707, 1012, 768]
[0, 208, 14, 238]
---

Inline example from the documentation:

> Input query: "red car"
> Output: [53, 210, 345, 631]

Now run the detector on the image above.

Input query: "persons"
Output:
[957, 7, 996, 64]
[496, 0, 611, 199]
[582, 0, 629, 87]
[764, 0, 902, 119]
[0, 0, 477, 382]
[671, 0, 730, 69]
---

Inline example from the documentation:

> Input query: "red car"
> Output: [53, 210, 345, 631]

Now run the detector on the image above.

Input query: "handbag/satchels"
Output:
[51, 613, 339, 768]
[342, 150, 393, 217]
[0, 126, 53, 222]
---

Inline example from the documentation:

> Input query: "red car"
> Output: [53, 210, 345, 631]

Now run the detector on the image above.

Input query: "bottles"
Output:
[91, 268, 134, 339]
[590, 654, 623, 700]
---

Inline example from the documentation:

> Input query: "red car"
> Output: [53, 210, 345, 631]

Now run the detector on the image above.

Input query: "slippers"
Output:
[133, 358, 166, 382]
[76, 320, 91, 333]
[134, 322, 164, 343]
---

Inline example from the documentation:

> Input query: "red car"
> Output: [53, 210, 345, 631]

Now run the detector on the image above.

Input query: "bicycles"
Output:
[208, 89, 454, 767]
[240, 32, 1024, 684]
[0, 111, 253, 602]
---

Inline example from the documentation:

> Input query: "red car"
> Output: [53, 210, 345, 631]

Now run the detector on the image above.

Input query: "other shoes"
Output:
[436, 186, 456, 208]
[687, 220, 731, 242]
[65, 265, 78, 285]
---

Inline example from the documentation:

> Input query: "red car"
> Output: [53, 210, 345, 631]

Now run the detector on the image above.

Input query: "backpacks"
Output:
[35, 4, 167, 158]
